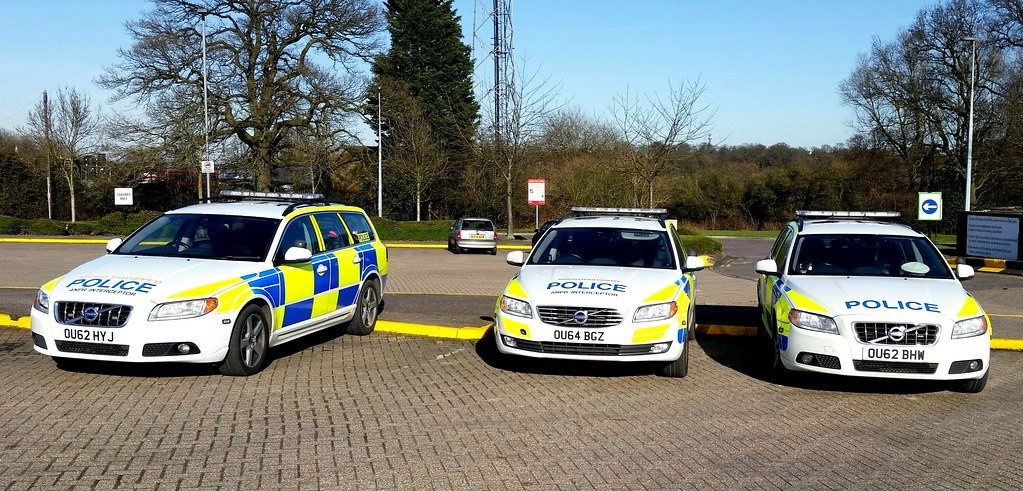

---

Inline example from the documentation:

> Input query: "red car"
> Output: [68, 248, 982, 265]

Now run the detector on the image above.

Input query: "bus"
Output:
[139, 168, 220, 188]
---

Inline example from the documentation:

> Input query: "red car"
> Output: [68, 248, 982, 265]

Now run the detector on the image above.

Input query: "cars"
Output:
[531, 219, 559, 249]
[756, 211, 993, 394]
[448, 218, 499, 255]
[494, 208, 717, 378]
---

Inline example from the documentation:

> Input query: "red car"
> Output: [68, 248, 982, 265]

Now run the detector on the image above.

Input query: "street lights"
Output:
[376, 87, 383, 218]
[197, 8, 211, 204]
[959, 37, 978, 212]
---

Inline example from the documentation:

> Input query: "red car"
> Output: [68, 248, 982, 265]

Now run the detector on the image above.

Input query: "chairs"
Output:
[877, 241, 902, 266]
[476, 223, 487, 229]
[802, 237, 827, 262]
[463, 222, 470, 229]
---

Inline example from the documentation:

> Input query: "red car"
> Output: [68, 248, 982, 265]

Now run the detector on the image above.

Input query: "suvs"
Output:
[30, 190, 390, 377]
